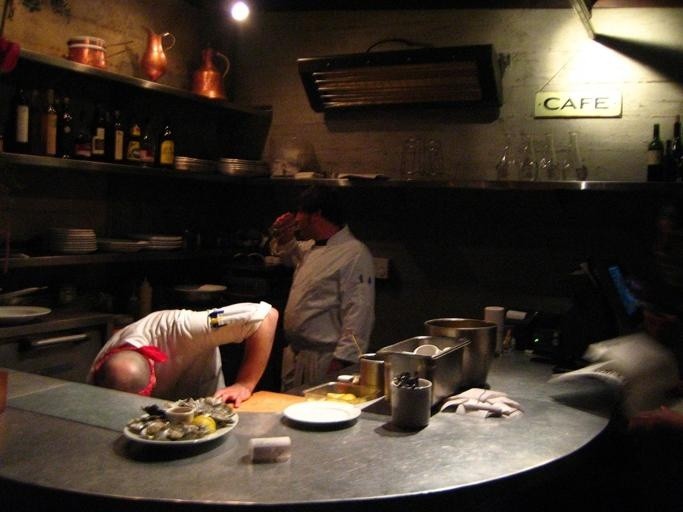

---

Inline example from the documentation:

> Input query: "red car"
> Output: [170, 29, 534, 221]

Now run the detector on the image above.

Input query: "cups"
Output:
[395, 134, 446, 181]
[268, 216, 301, 240]
[412, 343, 440, 358]
[165, 406, 194, 424]
[482, 306, 505, 358]
[390, 376, 432, 429]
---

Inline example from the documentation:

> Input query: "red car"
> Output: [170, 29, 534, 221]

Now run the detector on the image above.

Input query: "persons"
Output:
[629, 405, 682, 435]
[86, 301, 279, 409]
[269, 184, 375, 392]
[642, 307, 682, 385]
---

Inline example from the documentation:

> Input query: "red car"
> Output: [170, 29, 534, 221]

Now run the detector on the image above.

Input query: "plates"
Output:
[175, 154, 257, 177]
[1, 306, 53, 323]
[120, 410, 240, 447]
[282, 399, 364, 428]
[45, 224, 184, 253]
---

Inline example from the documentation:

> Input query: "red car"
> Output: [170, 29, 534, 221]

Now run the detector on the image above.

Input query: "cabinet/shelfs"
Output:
[1, 44, 272, 267]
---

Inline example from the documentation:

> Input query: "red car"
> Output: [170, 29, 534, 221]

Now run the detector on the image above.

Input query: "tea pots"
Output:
[133, 25, 176, 82]
[188, 41, 230, 102]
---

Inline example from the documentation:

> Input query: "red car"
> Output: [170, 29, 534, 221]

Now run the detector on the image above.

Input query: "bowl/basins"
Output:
[423, 318, 498, 388]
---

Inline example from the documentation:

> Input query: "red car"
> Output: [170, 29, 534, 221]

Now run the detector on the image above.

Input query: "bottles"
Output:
[646, 118, 683, 183]
[9, 73, 177, 167]
[138, 277, 153, 319]
[495, 131, 588, 181]
